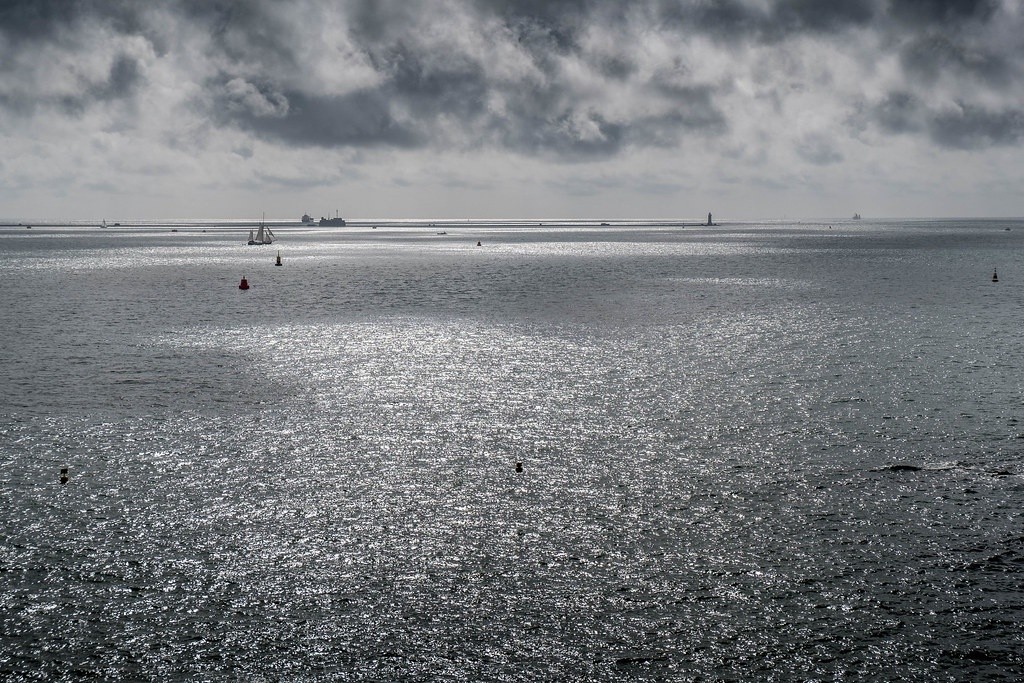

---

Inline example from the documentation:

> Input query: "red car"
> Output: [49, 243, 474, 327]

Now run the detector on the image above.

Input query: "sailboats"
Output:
[246, 213, 276, 245]
